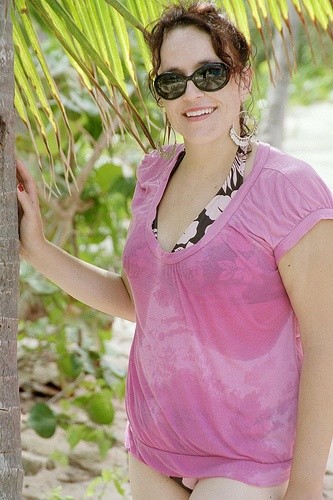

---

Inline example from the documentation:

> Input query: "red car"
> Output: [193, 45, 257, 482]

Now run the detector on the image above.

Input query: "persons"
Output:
[11, 0, 333, 500]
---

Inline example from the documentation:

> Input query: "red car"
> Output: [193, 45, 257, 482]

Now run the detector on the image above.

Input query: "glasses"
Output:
[155, 63, 242, 100]
